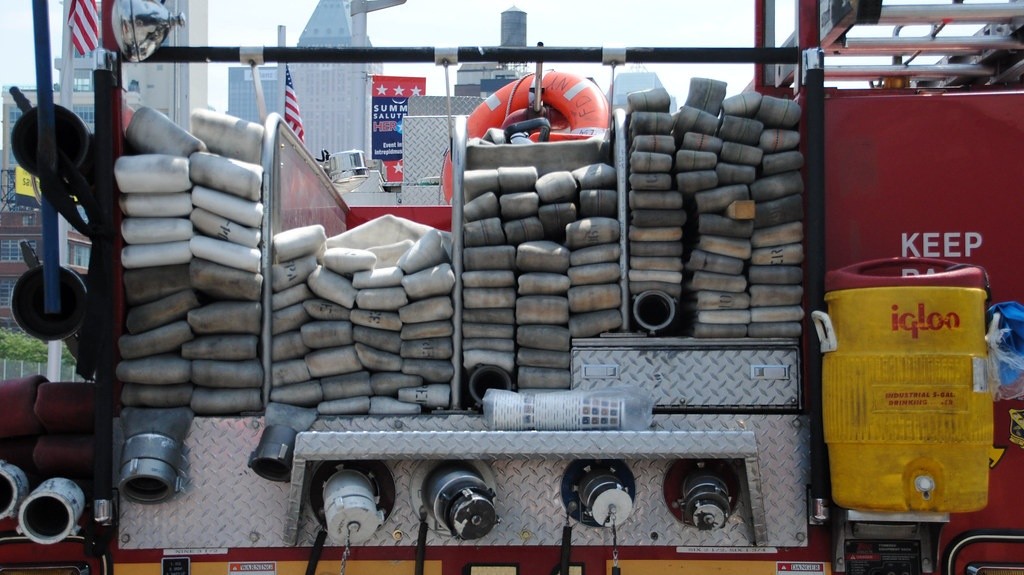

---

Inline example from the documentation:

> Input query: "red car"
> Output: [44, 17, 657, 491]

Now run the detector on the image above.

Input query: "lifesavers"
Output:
[442, 69, 608, 207]
